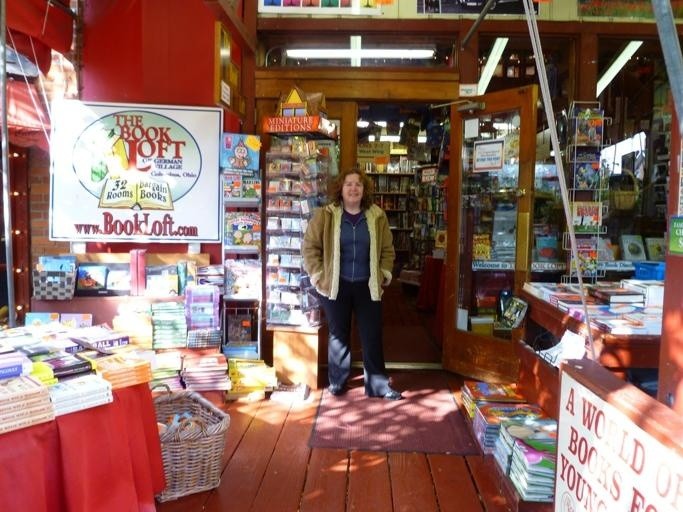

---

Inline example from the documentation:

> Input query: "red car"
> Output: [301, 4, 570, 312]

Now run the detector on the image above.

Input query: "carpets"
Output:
[308, 367, 482, 460]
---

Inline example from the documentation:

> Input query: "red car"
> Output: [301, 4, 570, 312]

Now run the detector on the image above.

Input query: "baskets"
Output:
[151, 383, 230, 504]
[603, 169, 639, 211]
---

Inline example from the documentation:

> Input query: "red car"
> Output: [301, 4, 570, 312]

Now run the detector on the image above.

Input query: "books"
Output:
[461, 380, 558, 503]
[0, 254, 232, 437]
[522, 275, 664, 336]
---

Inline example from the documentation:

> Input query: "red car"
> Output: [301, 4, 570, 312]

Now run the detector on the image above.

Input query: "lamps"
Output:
[282, 36, 435, 62]
[470, 35, 510, 96]
[593, 38, 644, 101]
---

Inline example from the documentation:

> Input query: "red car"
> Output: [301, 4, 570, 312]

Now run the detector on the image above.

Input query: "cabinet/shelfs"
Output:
[266, 139, 326, 393]
[363, 165, 447, 312]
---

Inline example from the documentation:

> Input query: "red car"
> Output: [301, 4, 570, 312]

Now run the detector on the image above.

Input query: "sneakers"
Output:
[329, 377, 346, 396]
[367, 384, 402, 400]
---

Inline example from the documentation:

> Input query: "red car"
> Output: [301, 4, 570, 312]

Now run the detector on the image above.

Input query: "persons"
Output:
[300, 167, 402, 400]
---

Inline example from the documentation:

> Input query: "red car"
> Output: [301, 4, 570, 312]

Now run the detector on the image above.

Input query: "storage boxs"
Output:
[30, 268, 76, 301]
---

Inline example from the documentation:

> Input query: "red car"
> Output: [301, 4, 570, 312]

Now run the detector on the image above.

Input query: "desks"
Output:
[0, 382, 167, 511]
[517, 282, 663, 383]
